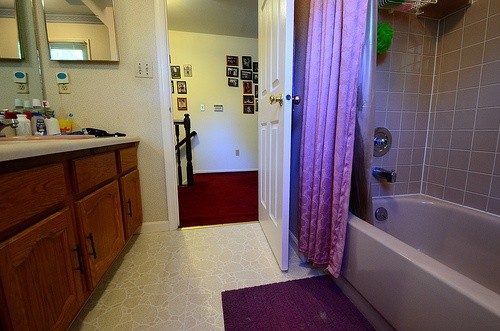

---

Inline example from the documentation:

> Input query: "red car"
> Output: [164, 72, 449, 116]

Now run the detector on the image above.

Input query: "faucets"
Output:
[0, 119, 18, 132]
[372, 167, 397, 183]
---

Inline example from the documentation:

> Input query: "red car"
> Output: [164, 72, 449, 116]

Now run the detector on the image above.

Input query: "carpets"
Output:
[220, 274, 377, 331]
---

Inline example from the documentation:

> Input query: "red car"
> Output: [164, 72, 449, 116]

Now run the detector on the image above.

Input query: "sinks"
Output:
[0, 135, 95, 143]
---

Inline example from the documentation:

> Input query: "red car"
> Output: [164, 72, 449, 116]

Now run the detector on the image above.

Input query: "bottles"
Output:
[0, 99, 80, 138]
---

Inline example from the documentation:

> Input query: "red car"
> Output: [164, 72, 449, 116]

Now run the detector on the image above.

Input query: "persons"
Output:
[226, 55, 259, 114]
[170, 66, 188, 110]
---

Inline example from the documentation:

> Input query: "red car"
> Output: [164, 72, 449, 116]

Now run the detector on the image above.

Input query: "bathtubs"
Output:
[330, 194, 500, 331]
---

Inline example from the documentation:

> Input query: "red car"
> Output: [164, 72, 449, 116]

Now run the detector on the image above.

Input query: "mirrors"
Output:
[0, 0, 50, 113]
[0, 0, 27, 59]
[43, 0, 121, 62]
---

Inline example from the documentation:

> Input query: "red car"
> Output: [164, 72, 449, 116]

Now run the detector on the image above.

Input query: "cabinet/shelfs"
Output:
[118, 146, 142, 240]
[0, 151, 126, 331]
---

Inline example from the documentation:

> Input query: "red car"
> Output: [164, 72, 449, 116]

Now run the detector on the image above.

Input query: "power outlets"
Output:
[58, 82, 71, 95]
[14, 84, 27, 93]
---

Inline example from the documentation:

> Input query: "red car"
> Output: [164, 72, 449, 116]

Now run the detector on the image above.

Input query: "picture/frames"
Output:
[170, 55, 193, 110]
[226, 55, 259, 114]
